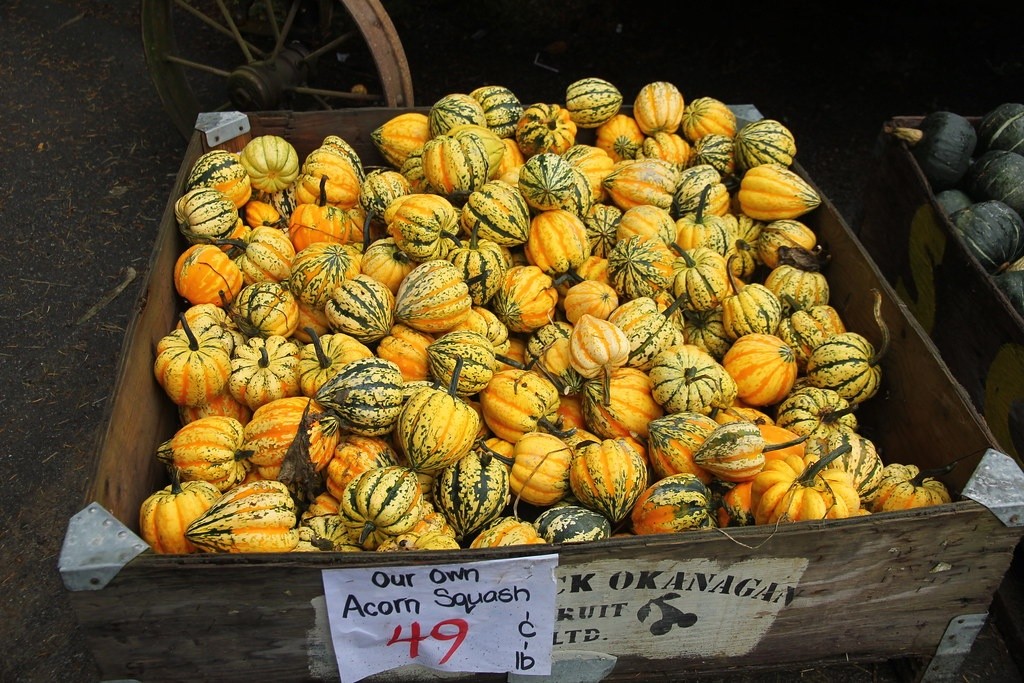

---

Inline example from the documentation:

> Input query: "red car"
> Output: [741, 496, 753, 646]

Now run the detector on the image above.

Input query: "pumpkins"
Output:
[134, 77, 1024, 557]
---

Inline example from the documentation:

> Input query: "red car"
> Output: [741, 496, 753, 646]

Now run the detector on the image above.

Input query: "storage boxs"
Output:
[53, 105, 1023, 683]
[857, 113, 1024, 673]
